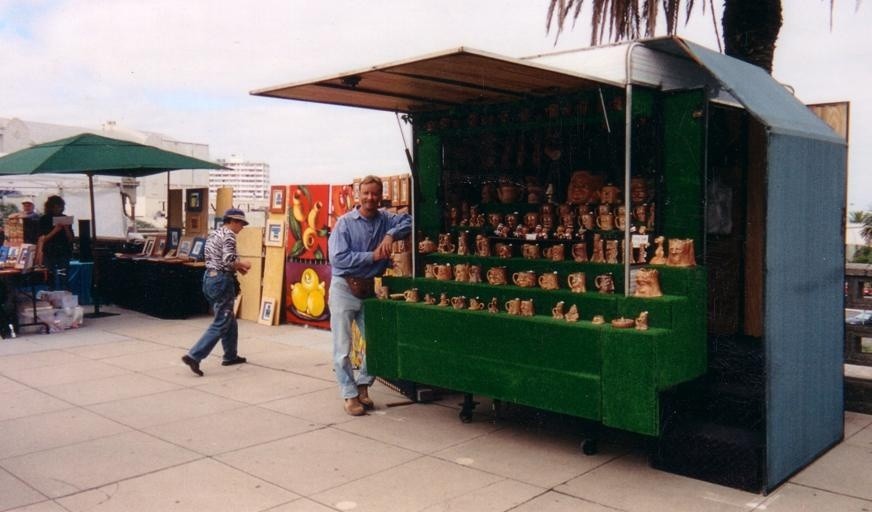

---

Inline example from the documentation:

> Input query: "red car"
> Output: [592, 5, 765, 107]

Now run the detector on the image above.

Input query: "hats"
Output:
[224, 208, 249, 225]
[21, 199, 34, 204]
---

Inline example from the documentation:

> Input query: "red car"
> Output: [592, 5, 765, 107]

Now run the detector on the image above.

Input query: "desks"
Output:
[69, 253, 207, 320]
[0, 266, 49, 339]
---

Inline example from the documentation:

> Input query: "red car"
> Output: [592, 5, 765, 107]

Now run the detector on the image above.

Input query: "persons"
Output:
[327, 175, 413, 416]
[181, 208, 252, 376]
[36, 195, 75, 293]
[8, 198, 40, 245]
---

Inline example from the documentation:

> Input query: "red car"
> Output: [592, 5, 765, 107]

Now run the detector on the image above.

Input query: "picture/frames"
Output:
[141, 228, 206, 260]
[264, 185, 286, 247]
[257, 296, 276, 326]
[186, 189, 202, 233]
[0, 243, 33, 269]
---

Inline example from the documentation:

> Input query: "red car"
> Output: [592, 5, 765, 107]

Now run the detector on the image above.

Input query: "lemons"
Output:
[291, 269, 326, 317]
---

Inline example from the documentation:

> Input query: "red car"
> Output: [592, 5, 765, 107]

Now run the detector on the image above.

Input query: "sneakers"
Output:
[183, 355, 204, 376]
[358, 384, 374, 408]
[221, 357, 246, 366]
[343, 398, 365, 416]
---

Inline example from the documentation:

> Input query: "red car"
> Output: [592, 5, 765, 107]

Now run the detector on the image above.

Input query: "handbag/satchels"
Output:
[344, 275, 374, 298]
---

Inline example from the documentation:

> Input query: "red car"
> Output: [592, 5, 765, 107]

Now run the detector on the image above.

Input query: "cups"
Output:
[376, 199, 650, 322]
